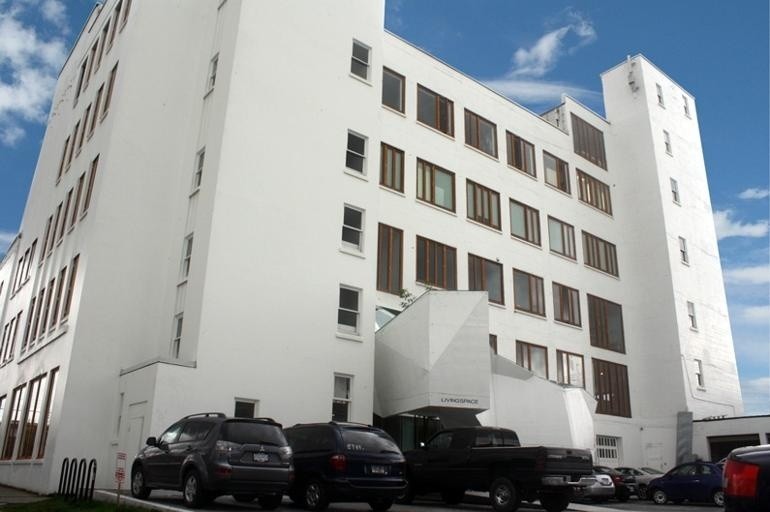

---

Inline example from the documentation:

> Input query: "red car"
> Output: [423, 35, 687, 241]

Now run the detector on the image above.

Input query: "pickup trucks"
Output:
[399, 424, 594, 511]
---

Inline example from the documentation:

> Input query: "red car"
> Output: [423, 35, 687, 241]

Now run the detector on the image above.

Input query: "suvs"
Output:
[125, 408, 302, 511]
[276, 421, 411, 511]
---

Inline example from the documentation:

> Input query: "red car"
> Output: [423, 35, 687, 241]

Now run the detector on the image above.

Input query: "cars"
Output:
[566, 444, 769, 511]
